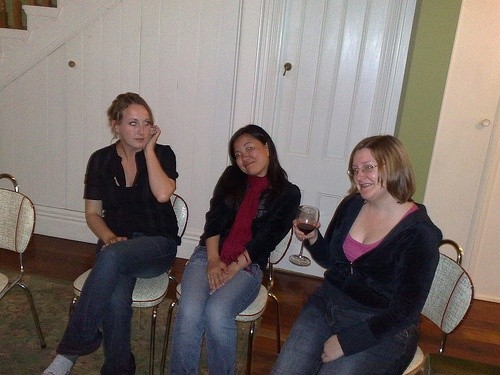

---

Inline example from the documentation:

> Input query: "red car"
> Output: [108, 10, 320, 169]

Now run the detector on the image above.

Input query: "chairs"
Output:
[402, 239, 474, 375]
[69, 195, 189, 375]
[0, 173, 47, 349]
[159, 198, 293, 375]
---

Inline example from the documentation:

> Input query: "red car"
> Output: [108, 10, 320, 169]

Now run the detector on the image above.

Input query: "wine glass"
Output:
[289, 206, 319, 266]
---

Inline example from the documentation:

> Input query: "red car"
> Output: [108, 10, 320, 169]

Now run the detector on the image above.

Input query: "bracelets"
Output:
[242, 253, 250, 266]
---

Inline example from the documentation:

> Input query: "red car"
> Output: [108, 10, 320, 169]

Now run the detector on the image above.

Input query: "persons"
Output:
[269, 133, 444, 375]
[39, 91, 180, 375]
[168, 124, 301, 375]
